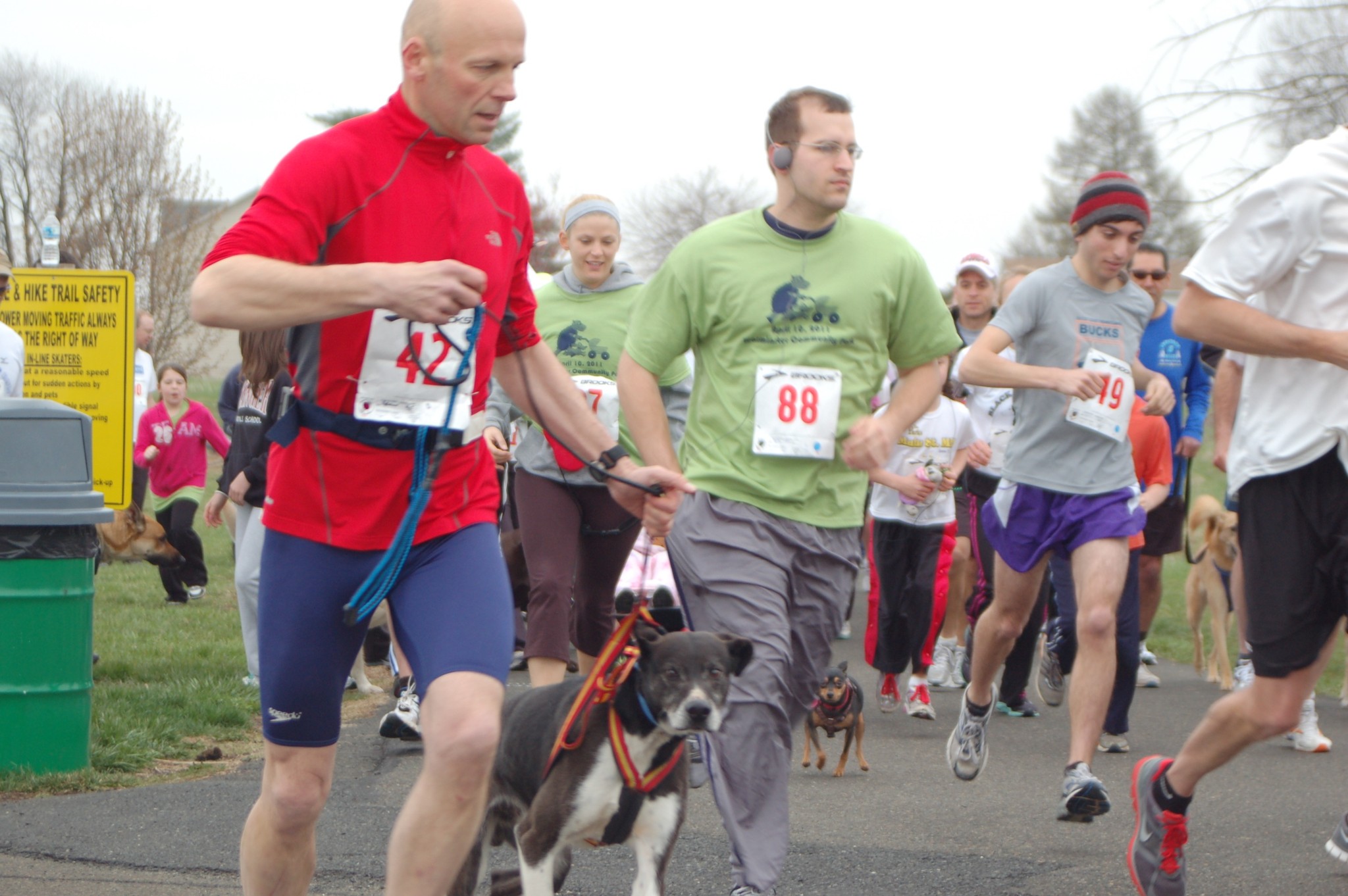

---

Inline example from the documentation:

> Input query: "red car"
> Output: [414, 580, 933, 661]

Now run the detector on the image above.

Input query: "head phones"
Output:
[767, 115, 792, 169]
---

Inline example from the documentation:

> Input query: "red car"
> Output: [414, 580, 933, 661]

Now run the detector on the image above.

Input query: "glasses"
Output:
[1132, 270, 1166, 281]
[798, 141, 862, 160]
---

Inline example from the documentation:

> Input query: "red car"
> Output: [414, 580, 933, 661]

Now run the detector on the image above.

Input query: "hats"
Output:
[957, 254, 996, 279]
[1071, 171, 1150, 236]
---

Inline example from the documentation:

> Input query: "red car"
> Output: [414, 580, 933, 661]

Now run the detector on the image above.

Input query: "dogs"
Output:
[800, 659, 870, 777]
[1186, 495, 1240, 691]
[95, 497, 186, 568]
[448, 617, 754, 896]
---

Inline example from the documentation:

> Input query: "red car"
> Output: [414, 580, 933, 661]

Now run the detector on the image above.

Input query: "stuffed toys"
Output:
[900, 459, 941, 516]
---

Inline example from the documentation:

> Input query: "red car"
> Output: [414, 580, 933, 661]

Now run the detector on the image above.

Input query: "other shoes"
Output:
[1135, 663, 1160, 688]
[1233, 659, 1257, 689]
[188, 585, 206, 599]
[729, 882, 776, 896]
[1286, 699, 1332, 753]
[926, 642, 954, 684]
[344, 675, 358, 690]
[837, 621, 851, 640]
[946, 651, 967, 687]
[996, 688, 1039, 717]
[1138, 641, 1156, 665]
[241, 674, 260, 686]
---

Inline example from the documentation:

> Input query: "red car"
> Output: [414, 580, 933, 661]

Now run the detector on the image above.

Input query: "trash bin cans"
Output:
[0, 398, 115, 769]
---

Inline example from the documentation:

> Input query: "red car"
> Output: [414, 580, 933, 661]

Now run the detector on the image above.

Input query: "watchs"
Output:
[589, 444, 631, 484]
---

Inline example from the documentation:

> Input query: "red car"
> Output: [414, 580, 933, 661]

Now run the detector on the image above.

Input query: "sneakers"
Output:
[876, 670, 901, 713]
[1057, 761, 1111, 822]
[1098, 731, 1129, 753]
[947, 682, 998, 781]
[1035, 635, 1063, 706]
[379, 676, 420, 741]
[1324, 813, 1348, 862]
[904, 684, 936, 719]
[1128, 755, 1189, 896]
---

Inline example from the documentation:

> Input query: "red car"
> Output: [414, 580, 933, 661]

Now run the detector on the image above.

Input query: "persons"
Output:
[190, 0, 691, 896]
[0, 85, 1348, 896]
